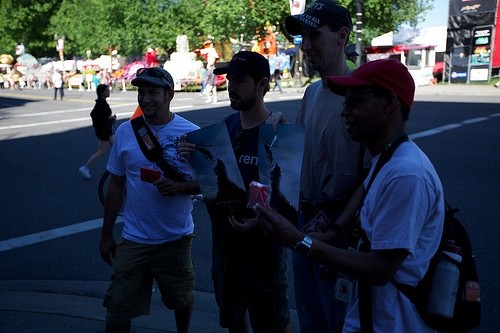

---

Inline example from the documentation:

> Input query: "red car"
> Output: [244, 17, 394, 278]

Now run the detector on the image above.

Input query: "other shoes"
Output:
[79, 166, 91, 178]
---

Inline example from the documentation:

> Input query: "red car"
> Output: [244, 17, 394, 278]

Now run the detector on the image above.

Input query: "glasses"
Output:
[342, 90, 380, 107]
[232, 55, 251, 66]
[136, 68, 172, 85]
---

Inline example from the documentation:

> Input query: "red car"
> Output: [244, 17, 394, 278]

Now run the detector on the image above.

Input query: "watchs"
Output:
[294, 233, 313, 256]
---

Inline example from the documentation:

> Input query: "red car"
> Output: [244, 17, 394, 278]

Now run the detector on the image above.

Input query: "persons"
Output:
[225, 58, 446, 333]
[263, 0, 374, 333]
[51, 68, 65, 101]
[97, 67, 202, 333]
[0, 43, 114, 93]
[78, 84, 117, 179]
[194, 40, 315, 104]
[176, 50, 289, 333]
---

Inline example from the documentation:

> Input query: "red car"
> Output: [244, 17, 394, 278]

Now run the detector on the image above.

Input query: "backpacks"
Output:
[353, 136, 481, 333]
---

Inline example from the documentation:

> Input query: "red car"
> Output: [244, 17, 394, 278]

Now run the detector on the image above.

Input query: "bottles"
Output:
[428, 239, 463, 319]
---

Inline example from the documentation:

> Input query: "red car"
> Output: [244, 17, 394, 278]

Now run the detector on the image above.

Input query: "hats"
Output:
[327, 58, 415, 108]
[131, 67, 174, 91]
[285, 0, 353, 36]
[213, 50, 270, 79]
